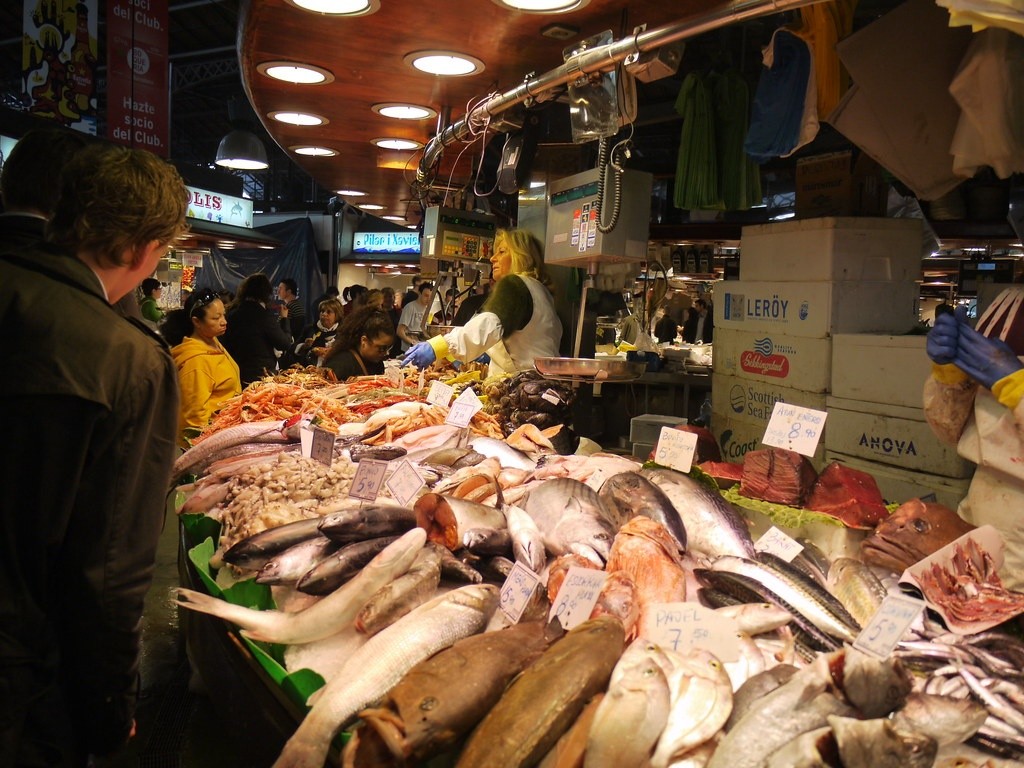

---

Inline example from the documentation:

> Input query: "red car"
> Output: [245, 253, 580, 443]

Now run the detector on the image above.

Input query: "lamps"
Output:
[214, 128, 270, 170]
[625, 40, 686, 84]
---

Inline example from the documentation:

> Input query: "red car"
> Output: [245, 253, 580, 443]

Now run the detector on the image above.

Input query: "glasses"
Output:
[189, 292, 220, 318]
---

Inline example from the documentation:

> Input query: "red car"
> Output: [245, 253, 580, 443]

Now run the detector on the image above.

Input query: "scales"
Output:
[533, 166, 654, 444]
[420, 206, 498, 337]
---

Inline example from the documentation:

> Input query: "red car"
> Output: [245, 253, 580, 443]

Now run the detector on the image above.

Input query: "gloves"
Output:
[400, 333, 449, 372]
[924, 304, 1024, 391]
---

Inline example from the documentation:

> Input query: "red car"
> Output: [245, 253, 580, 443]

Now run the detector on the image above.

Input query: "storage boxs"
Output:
[739, 215, 924, 281]
[832, 335, 936, 410]
[629, 413, 688, 445]
[713, 328, 834, 393]
[825, 396, 977, 480]
[824, 451, 972, 514]
[712, 281, 921, 336]
[713, 373, 826, 445]
[632, 443, 653, 462]
[710, 413, 826, 475]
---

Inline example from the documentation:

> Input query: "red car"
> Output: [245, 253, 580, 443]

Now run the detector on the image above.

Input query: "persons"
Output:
[923, 302, 1024, 600]
[651, 299, 714, 345]
[401, 230, 563, 396]
[0, 148, 191, 768]
[170, 287, 242, 602]
[138, 273, 463, 392]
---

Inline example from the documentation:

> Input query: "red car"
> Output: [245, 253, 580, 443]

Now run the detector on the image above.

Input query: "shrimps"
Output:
[186, 382, 366, 446]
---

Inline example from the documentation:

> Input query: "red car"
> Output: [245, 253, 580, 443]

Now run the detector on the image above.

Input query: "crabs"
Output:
[361, 402, 503, 446]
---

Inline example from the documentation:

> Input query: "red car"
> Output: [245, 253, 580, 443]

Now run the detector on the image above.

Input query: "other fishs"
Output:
[825, 557, 887, 627]
[606, 635, 675, 685]
[352, 545, 442, 634]
[650, 646, 733, 768]
[693, 492, 1024, 768]
[531, 692, 605, 768]
[458, 613, 626, 768]
[297, 535, 401, 597]
[165, 422, 688, 636]
[597, 473, 688, 556]
[583, 657, 669, 767]
[340, 582, 566, 768]
[638, 601, 765, 686]
[168, 505, 427, 647]
[642, 465, 753, 558]
[272, 583, 502, 768]
